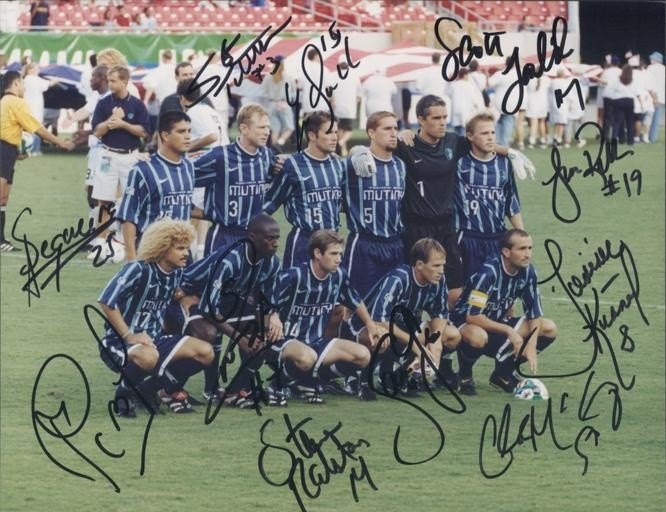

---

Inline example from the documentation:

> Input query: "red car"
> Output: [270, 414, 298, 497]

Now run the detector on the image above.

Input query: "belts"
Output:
[102, 145, 137, 154]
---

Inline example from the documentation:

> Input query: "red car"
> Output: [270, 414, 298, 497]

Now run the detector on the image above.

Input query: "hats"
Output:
[650, 51, 663, 63]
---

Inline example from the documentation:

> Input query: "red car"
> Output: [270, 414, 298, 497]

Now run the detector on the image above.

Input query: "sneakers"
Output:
[577, 139, 587, 147]
[633, 136, 640, 144]
[322, 379, 351, 394]
[0, 241, 22, 252]
[186, 391, 205, 406]
[539, 142, 546, 149]
[344, 376, 359, 394]
[159, 388, 196, 414]
[412, 375, 434, 391]
[136, 381, 166, 416]
[436, 370, 458, 388]
[488, 370, 521, 394]
[642, 135, 650, 145]
[202, 387, 225, 402]
[357, 383, 377, 401]
[114, 385, 138, 418]
[527, 140, 536, 149]
[553, 137, 557, 146]
[295, 382, 325, 404]
[222, 391, 263, 409]
[556, 141, 563, 149]
[457, 376, 477, 395]
[86, 249, 102, 260]
[517, 143, 525, 149]
[564, 143, 570, 149]
[264, 386, 288, 407]
[377, 375, 398, 396]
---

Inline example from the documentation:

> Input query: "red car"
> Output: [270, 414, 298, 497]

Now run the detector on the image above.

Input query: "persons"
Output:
[177, 78, 225, 261]
[142, 50, 179, 107]
[198, 49, 247, 146]
[468, 60, 487, 94]
[329, 52, 360, 157]
[62, 49, 140, 129]
[103, 9, 117, 26]
[526, 67, 550, 149]
[257, 61, 294, 154]
[40, 106, 59, 147]
[209, 0, 234, 11]
[450, 70, 486, 133]
[97, 219, 214, 418]
[117, 110, 205, 405]
[562, 78, 585, 149]
[516, 100, 528, 150]
[542, 17, 555, 29]
[641, 60, 657, 144]
[628, 55, 646, 144]
[114, 5, 131, 26]
[518, 16, 534, 32]
[599, 57, 626, 145]
[416, 52, 451, 120]
[21, 63, 58, 155]
[345, 239, 462, 402]
[0, 71, 74, 249]
[88, 67, 151, 263]
[485, 54, 519, 149]
[86, 66, 108, 235]
[397, 114, 525, 295]
[348, 94, 536, 390]
[134, 103, 341, 402]
[275, 110, 412, 393]
[296, 48, 333, 113]
[548, 66, 568, 148]
[173, 214, 281, 410]
[129, 13, 144, 28]
[450, 228, 557, 398]
[266, 230, 390, 404]
[248, 0, 271, 9]
[144, 4, 156, 28]
[356, 67, 399, 116]
[158, 62, 214, 141]
[262, 111, 349, 270]
[596, 55, 614, 138]
[590, 69, 644, 146]
[650, 52, 666, 143]
[80, 53, 96, 105]
[31, 1, 49, 32]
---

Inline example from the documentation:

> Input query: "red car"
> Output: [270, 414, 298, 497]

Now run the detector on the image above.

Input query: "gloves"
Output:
[351, 145, 378, 179]
[507, 147, 536, 182]
